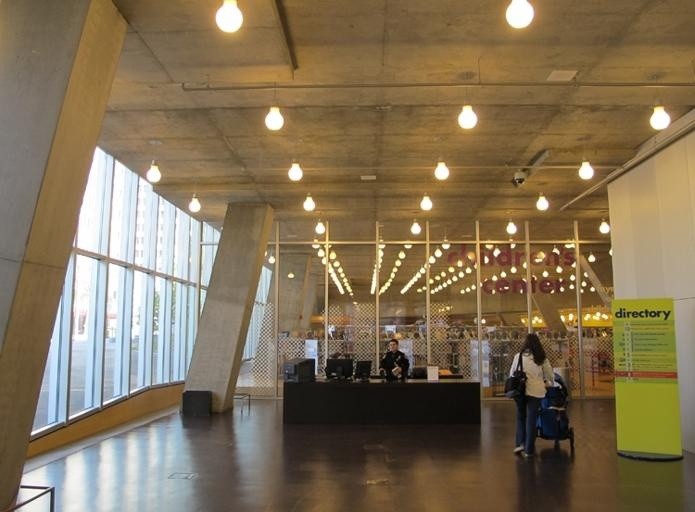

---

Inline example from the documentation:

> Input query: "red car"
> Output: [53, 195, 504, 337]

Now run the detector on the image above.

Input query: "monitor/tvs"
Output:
[326, 358, 353, 381]
[355, 361, 373, 381]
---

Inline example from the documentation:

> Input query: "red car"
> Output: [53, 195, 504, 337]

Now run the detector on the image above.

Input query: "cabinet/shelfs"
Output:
[334, 323, 613, 387]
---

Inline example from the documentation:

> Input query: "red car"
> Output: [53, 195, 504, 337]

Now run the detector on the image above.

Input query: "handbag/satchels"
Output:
[504, 377, 521, 398]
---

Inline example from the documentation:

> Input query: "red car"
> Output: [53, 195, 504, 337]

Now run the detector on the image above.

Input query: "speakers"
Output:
[183, 390, 212, 416]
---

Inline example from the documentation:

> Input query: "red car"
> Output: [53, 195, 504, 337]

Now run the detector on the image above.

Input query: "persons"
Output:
[508, 333, 555, 459]
[381, 339, 409, 383]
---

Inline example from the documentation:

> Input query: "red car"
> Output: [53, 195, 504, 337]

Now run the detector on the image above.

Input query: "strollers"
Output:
[532, 372, 577, 447]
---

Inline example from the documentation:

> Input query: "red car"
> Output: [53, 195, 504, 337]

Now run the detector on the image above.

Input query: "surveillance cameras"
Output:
[515, 172, 527, 185]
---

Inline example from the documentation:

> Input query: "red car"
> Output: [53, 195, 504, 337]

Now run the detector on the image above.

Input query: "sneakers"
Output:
[513, 446, 525, 453]
[524, 454, 532, 457]
